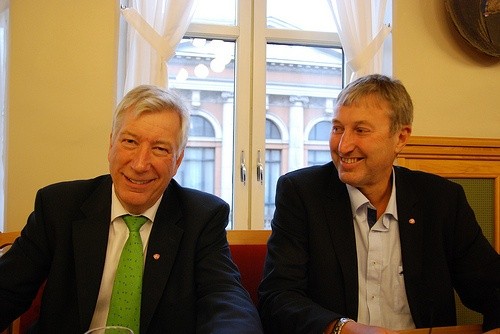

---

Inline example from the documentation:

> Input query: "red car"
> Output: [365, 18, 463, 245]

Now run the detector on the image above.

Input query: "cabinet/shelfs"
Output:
[394, 137, 500, 327]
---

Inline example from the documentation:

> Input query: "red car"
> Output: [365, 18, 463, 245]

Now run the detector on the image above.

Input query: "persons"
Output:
[262, 73, 500, 334]
[1, 86, 262, 334]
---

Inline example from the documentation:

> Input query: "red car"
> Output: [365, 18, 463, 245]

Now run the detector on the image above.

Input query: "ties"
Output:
[105, 215, 149, 334]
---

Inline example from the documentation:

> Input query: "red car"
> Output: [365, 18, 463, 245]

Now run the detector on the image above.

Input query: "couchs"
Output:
[228, 244, 268, 300]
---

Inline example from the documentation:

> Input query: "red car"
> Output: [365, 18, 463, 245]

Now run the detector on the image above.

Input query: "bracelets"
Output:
[332, 318, 356, 334]
